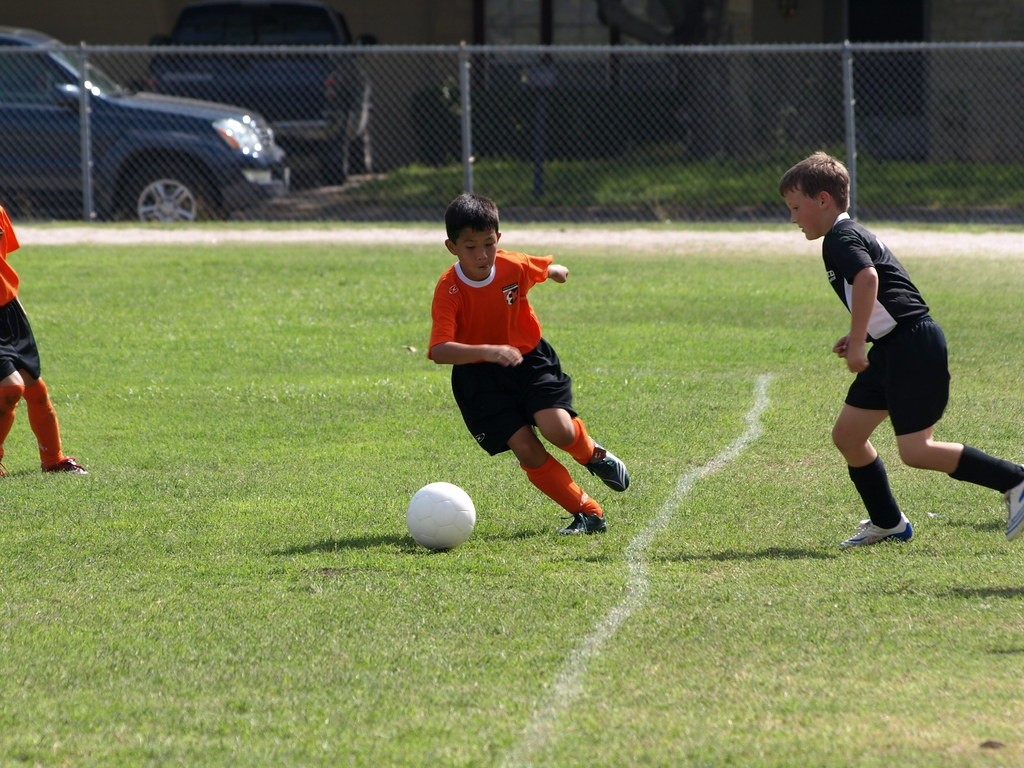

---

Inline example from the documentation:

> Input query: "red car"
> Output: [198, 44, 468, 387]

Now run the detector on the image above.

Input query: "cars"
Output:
[0, 25, 291, 225]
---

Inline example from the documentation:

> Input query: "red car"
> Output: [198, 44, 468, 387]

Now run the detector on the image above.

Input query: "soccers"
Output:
[405, 481, 477, 551]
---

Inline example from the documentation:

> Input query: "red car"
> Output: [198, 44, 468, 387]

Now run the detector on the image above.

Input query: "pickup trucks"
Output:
[122, 0, 386, 189]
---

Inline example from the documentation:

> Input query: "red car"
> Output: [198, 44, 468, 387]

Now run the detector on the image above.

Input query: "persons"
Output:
[778, 149, 1024, 547]
[426, 193, 630, 533]
[0, 204, 89, 473]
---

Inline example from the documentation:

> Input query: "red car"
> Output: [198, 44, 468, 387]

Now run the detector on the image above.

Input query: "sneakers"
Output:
[841, 512, 913, 549]
[1002, 464, 1024, 541]
[42, 458, 87, 474]
[582, 437, 629, 492]
[559, 512, 608, 535]
